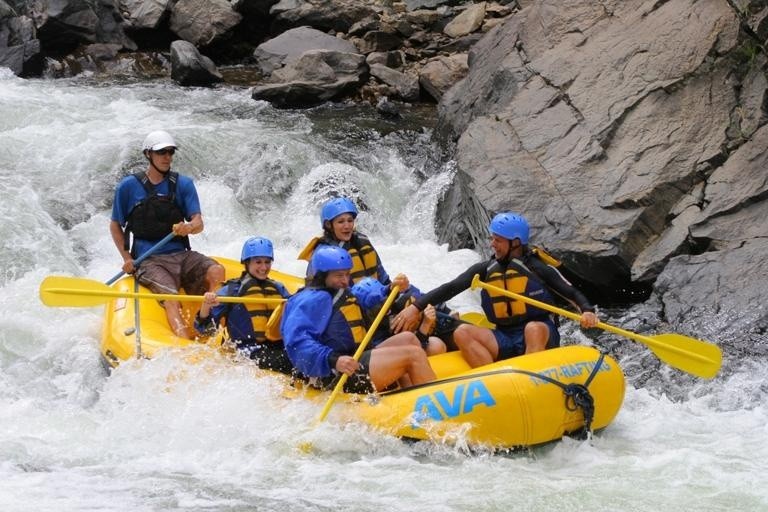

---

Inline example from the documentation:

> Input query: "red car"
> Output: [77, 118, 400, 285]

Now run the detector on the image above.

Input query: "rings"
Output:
[398, 318, 402, 320]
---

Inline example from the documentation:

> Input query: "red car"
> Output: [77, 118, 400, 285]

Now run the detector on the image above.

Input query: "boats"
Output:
[102, 255, 628, 455]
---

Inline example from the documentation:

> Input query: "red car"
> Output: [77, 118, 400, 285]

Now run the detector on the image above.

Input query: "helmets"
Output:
[142, 131, 178, 153]
[241, 198, 387, 310]
[489, 213, 528, 246]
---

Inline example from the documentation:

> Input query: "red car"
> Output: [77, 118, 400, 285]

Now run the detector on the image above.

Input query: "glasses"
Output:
[157, 149, 175, 155]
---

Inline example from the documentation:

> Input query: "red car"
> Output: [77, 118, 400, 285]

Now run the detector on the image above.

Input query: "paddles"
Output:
[38, 275, 290, 307]
[297, 285, 401, 454]
[470, 274, 722, 380]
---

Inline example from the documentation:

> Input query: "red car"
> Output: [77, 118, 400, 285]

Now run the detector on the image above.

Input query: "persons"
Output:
[280, 246, 437, 393]
[193, 237, 293, 374]
[110, 130, 226, 338]
[391, 212, 600, 369]
[352, 276, 465, 357]
[313, 198, 391, 289]
